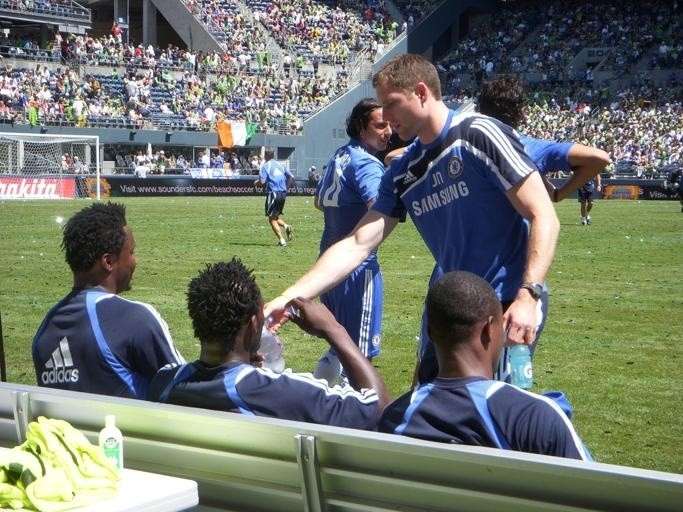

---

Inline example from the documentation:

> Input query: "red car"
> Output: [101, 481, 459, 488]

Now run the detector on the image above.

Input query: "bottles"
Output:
[509, 344, 533, 389]
[257, 325, 286, 374]
[98, 414, 124, 471]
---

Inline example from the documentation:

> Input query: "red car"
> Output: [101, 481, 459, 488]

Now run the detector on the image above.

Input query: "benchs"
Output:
[0, 378, 683, 511]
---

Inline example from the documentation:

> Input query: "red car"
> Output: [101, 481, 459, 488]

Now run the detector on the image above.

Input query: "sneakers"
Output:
[580, 214, 592, 226]
[277, 224, 294, 247]
